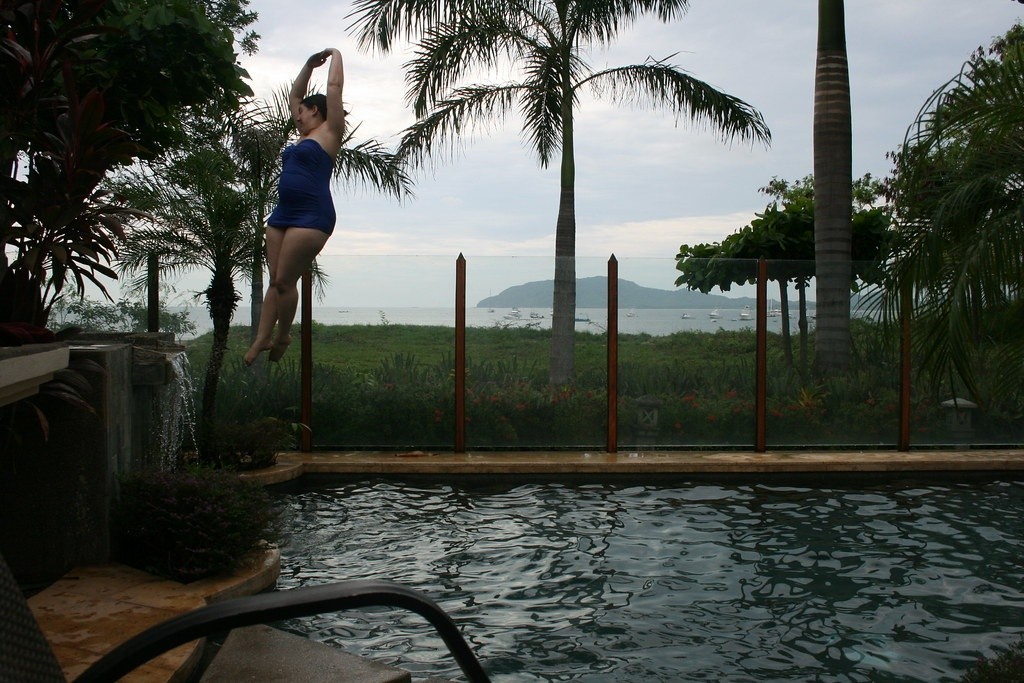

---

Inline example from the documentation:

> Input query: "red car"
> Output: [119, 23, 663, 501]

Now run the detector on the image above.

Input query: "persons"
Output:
[243, 50, 344, 366]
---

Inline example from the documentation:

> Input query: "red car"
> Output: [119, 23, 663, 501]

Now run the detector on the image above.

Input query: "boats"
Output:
[767, 297, 816, 320]
[627, 311, 638, 317]
[709, 308, 721, 319]
[682, 312, 693, 320]
[487, 306, 591, 324]
[739, 305, 752, 320]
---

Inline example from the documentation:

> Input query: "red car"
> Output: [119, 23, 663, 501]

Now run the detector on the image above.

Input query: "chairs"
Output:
[0, 558, 491, 683]
